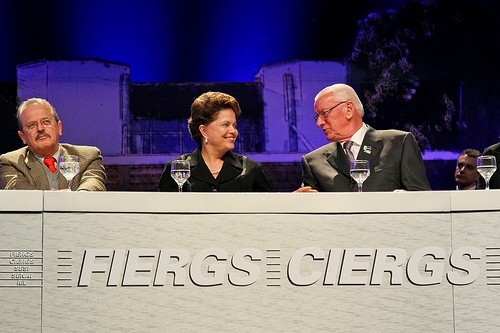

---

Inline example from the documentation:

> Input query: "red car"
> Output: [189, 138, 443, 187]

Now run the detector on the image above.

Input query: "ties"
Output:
[43, 156, 57, 173]
[342, 141, 355, 162]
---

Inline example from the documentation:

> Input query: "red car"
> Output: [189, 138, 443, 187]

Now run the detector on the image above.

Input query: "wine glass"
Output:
[350, 160, 370, 192]
[170, 160, 190, 192]
[59, 156, 80, 191]
[477, 156, 497, 190]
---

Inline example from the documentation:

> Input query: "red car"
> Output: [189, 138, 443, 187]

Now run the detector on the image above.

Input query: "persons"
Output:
[454, 141, 500, 190]
[293, 83, 431, 192]
[0, 98, 107, 191]
[156, 91, 273, 192]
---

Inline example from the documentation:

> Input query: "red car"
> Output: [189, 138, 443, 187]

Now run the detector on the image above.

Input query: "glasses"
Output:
[23, 119, 53, 129]
[314, 102, 344, 123]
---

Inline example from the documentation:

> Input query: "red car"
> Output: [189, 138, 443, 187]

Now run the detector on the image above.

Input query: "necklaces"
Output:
[211, 169, 221, 174]
